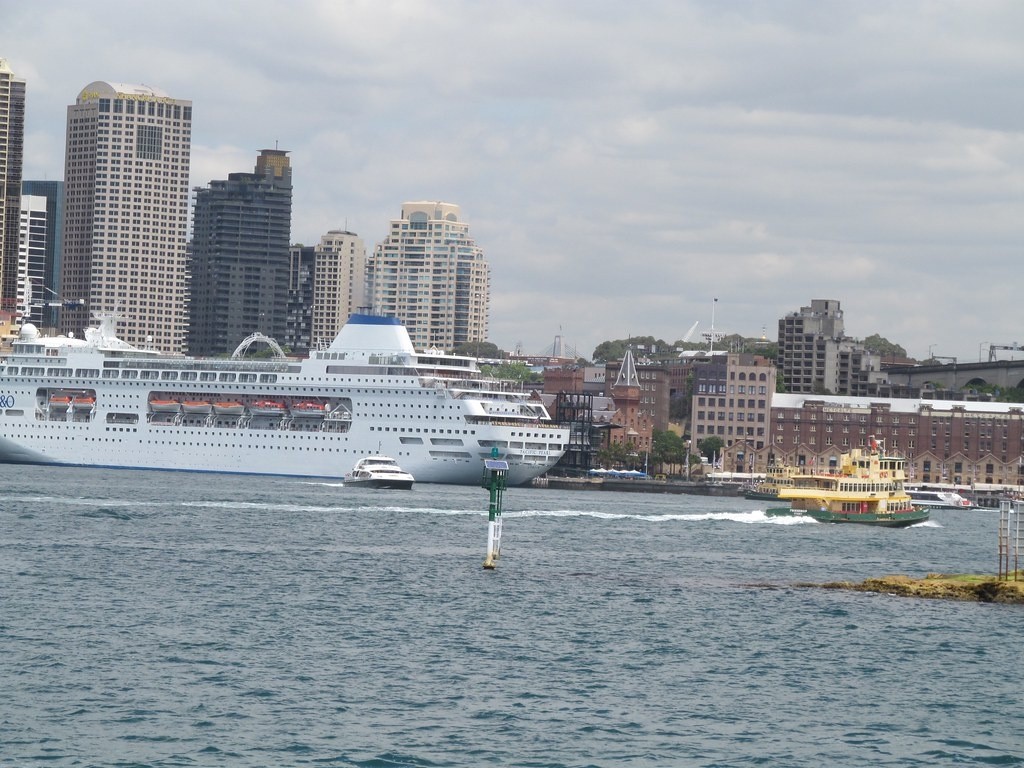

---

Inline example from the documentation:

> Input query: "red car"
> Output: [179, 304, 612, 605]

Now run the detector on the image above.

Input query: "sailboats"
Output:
[737, 450, 762, 496]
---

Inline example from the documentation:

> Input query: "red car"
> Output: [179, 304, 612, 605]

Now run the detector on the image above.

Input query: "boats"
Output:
[343, 456, 415, 492]
[904, 490, 981, 512]
[0, 304, 572, 486]
[745, 459, 800, 502]
[776, 437, 929, 529]
